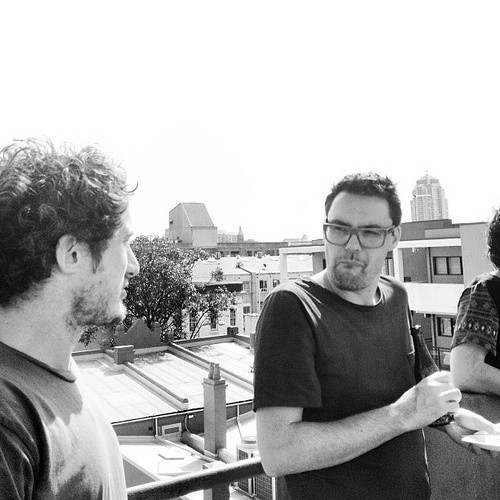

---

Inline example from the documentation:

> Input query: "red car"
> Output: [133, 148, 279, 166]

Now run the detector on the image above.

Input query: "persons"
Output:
[450, 206, 500, 397]
[253, 172, 500, 500]
[0, 133, 140, 500]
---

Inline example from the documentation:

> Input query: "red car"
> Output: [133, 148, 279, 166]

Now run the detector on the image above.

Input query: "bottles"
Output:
[409, 325, 451, 428]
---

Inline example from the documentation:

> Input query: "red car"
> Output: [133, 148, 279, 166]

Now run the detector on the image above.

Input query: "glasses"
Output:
[323, 222, 395, 249]
